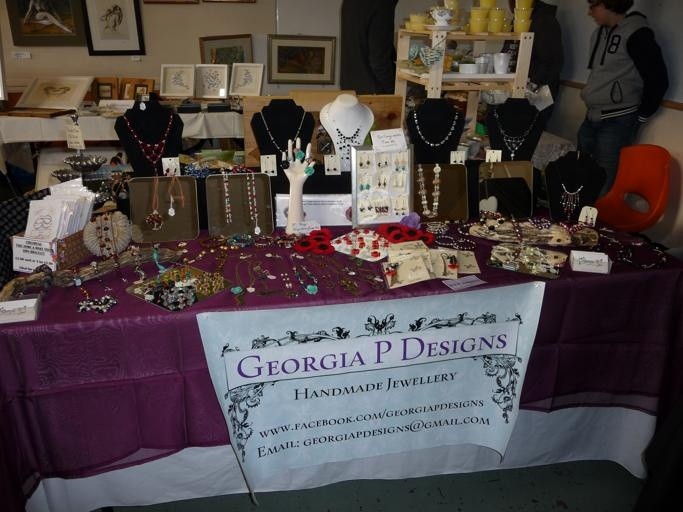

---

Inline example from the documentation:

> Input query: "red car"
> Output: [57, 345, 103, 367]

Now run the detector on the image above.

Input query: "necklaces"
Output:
[61, 101, 667, 313]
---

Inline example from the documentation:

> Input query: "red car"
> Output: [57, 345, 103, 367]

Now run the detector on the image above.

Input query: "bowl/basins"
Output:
[48, 155, 107, 182]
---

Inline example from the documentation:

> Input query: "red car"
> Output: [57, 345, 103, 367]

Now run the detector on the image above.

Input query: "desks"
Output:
[0, 221, 681, 509]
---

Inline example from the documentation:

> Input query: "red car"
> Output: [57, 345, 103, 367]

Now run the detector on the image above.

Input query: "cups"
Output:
[467, 0, 533, 33]
[458, 52, 510, 75]
[403, 0, 462, 29]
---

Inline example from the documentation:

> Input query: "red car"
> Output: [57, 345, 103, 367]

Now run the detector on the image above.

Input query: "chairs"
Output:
[593, 143, 673, 234]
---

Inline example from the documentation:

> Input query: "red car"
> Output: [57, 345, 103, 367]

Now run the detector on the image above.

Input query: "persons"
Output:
[573, 1, 669, 181]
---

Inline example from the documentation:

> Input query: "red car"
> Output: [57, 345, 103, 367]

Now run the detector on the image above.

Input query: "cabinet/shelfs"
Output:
[392, 25, 534, 167]
